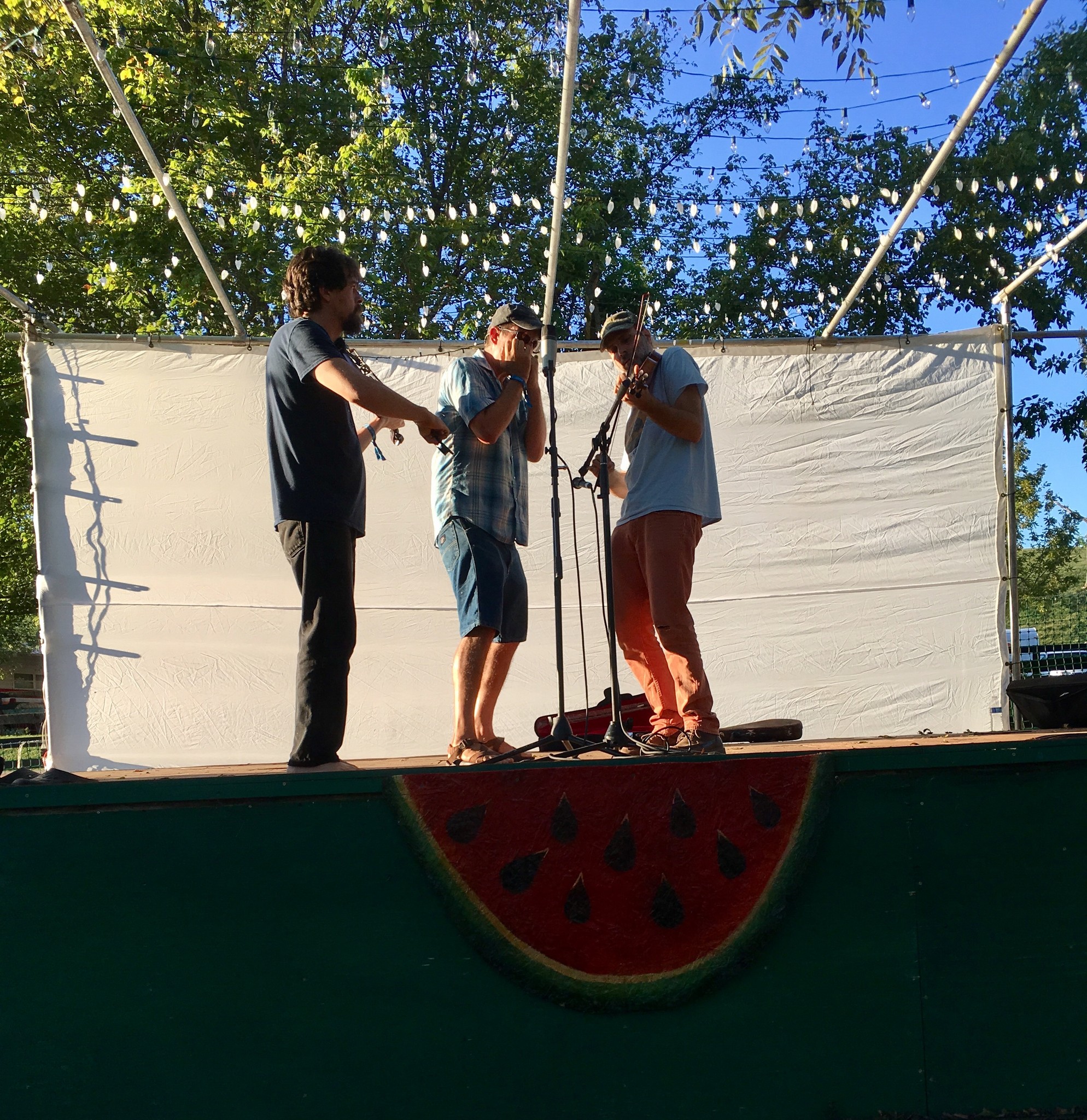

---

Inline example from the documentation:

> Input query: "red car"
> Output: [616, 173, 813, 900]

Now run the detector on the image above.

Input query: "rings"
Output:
[518, 345, 524, 349]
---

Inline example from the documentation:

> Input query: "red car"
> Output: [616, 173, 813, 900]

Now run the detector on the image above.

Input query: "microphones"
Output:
[572, 477, 592, 489]
[539, 324, 557, 380]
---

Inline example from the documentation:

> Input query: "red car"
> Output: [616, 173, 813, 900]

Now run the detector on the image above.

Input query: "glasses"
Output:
[499, 329, 541, 352]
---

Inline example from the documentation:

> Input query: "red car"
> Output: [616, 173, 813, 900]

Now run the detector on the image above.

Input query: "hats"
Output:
[600, 311, 640, 352]
[488, 304, 545, 330]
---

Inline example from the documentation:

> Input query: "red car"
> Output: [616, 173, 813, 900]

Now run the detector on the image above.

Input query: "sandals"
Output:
[480, 737, 535, 761]
[446, 739, 513, 765]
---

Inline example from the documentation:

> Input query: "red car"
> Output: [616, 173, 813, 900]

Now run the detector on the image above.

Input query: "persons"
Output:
[265, 244, 450, 775]
[431, 303, 546, 767]
[588, 311, 728, 758]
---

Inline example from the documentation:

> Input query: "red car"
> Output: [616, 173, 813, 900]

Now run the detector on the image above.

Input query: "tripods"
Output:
[478, 360, 721, 766]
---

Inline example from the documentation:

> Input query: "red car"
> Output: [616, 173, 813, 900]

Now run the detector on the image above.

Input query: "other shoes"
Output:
[664, 729, 726, 755]
[627, 727, 686, 750]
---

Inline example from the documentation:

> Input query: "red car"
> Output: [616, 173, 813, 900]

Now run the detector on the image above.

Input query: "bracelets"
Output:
[362, 423, 386, 461]
[510, 375, 532, 409]
